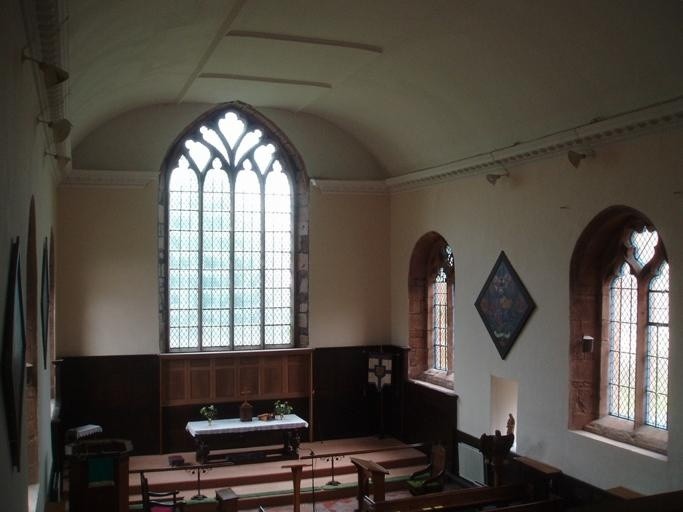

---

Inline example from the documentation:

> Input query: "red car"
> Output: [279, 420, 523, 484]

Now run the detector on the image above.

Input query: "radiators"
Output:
[457, 442, 484, 488]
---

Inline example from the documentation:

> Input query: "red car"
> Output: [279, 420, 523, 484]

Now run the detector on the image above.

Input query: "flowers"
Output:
[199, 405, 217, 426]
[272, 400, 294, 419]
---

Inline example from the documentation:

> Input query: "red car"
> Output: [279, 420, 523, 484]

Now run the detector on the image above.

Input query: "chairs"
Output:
[139, 470, 186, 511]
[404, 443, 449, 495]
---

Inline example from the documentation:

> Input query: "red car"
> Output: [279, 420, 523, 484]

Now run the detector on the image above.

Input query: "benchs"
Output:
[349, 457, 565, 512]
[476, 475, 592, 512]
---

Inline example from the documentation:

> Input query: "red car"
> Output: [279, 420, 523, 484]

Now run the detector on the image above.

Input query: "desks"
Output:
[185, 412, 310, 460]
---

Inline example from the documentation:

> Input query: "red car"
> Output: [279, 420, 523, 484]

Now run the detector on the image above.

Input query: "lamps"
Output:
[34, 114, 72, 143]
[566, 149, 594, 170]
[485, 172, 508, 186]
[20, 48, 69, 88]
[41, 149, 72, 170]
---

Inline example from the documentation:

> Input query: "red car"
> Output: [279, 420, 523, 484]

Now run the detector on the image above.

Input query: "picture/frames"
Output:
[1, 236, 25, 478]
[471, 248, 537, 360]
